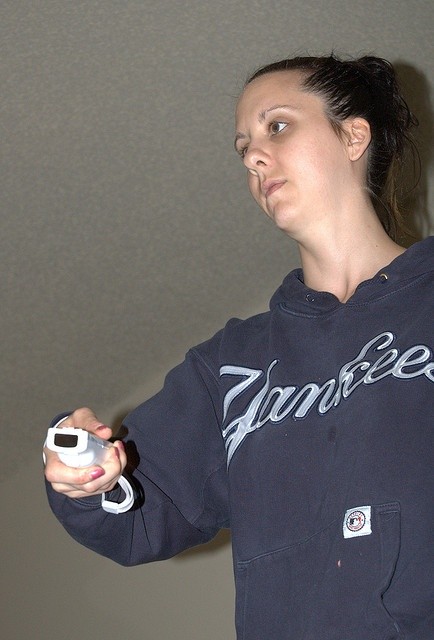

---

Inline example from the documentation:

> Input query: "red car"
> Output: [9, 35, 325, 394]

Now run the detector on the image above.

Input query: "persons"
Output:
[42, 54, 434, 639]
